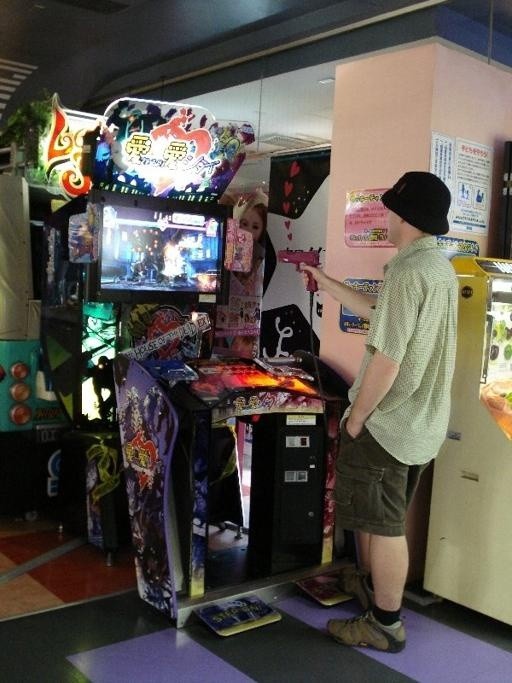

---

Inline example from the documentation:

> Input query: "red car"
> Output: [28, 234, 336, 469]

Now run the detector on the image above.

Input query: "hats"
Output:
[380, 170, 452, 235]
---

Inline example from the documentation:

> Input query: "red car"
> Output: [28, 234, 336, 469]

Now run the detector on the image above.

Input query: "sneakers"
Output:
[326, 607, 408, 654]
[336, 571, 376, 611]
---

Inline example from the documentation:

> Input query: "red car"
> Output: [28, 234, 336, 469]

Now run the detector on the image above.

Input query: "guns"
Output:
[276, 245, 323, 292]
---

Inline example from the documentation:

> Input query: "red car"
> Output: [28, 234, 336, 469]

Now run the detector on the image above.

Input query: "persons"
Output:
[300, 170, 461, 655]
[240, 203, 266, 240]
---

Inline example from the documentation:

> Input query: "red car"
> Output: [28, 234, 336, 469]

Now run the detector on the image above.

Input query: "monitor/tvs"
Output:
[88, 192, 234, 305]
